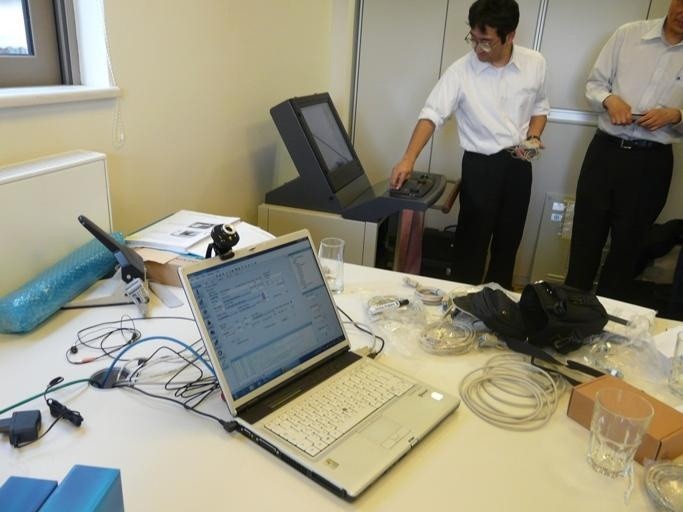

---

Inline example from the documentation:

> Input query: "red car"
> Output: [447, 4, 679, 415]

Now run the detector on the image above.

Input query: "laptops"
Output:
[176, 228, 461, 503]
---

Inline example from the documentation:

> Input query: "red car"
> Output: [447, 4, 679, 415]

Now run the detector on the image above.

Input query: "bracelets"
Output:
[527, 135, 540, 141]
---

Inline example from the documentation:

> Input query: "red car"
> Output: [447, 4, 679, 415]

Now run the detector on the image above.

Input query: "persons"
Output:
[389, 0, 551, 292]
[563, 1, 683, 299]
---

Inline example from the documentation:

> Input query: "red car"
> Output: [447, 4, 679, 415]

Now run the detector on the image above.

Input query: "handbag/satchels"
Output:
[453, 278, 608, 356]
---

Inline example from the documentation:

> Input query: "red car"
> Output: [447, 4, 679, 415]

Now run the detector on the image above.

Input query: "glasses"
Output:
[463, 30, 505, 54]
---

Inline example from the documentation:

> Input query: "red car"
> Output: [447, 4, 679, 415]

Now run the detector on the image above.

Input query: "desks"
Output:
[0, 253, 683, 511]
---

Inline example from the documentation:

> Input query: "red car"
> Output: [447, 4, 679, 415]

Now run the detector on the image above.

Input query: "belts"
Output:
[596, 127, 672, 151]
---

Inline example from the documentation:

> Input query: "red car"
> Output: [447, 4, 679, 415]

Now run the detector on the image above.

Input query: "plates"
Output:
[640, 460, 683, 512]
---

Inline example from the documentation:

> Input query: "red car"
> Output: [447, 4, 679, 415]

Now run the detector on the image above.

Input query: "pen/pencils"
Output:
[370, 299, 409, 315]
[607, 314, 630, 326]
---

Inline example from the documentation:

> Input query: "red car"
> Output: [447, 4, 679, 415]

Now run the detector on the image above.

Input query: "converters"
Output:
[0, 410, 41, 447]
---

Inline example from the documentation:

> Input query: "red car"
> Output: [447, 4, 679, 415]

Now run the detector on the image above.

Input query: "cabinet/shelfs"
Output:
[257, 201, 402, 270]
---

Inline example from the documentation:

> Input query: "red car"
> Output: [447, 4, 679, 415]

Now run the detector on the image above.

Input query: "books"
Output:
[123, 209, 276, 260]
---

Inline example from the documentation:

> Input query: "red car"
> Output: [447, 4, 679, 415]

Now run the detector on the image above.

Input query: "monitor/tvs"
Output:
[265, 91, 371, 212]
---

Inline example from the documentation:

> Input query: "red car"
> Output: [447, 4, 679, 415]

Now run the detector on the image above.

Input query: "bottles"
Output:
[315, 236, 345, 295]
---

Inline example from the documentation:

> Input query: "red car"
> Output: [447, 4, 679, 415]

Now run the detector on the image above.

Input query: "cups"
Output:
[666, 330, 683, 397]
[586, 387, 655, 478]
